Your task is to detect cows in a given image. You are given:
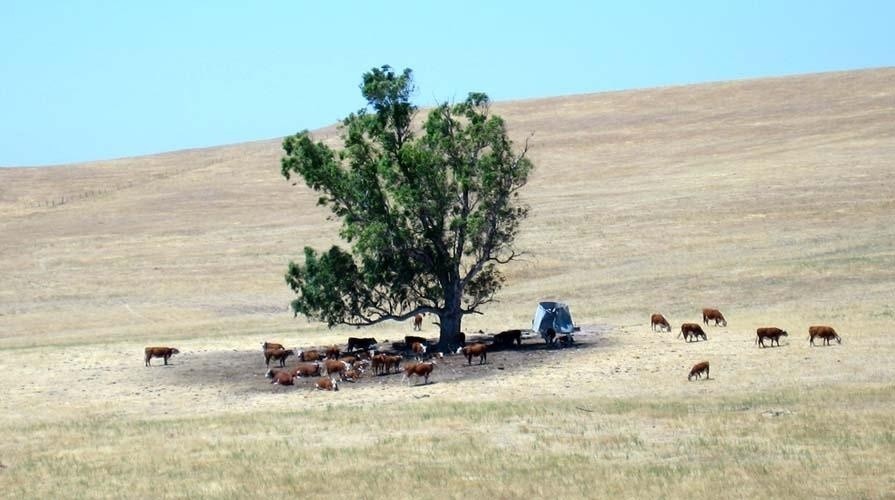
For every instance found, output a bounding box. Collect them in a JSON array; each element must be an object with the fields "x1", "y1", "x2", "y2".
[
  {"x1": 754, "y1": 327, "x2": 788, "y2": 348},
  {"x1": 677, "y1": 323, "x2": 707, "y2": 343},
  {"x1": 687, "y1": 361, "x2": 710, "y2": 382},
  {"x1": 544, "y1": 328, "x2": 555, "y2": 346},
  {"x1": 410, "y1": 341, "x2": 429, "y2": 361},
  {"x1": 401, "y1": 361, "x2": 435, "y2": 386},
  {"x1": 412, "y1": 313, "x2": 423, "y2": 331},
  {"x1": 807, "y1": 326, "x2": 841, "y2": 346},
  {"x1": 259, "y1": 336, "x2": 405, "y2": 390},
  {"x1": 463, "y1": 341, "x2": 488, "y2": 365},
  {"x1": 702, "y1": 308, "x2": 727, "y2": 328},
  {"x1": 144, "y1": 346, "x2": 179, "y2": 368},
  {"x1": 651, "y1": 313, "x2": 672, "y2": 332}
]
